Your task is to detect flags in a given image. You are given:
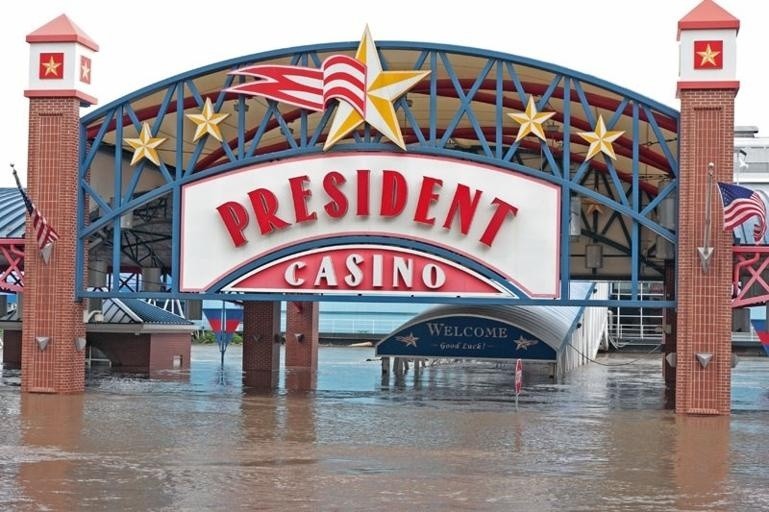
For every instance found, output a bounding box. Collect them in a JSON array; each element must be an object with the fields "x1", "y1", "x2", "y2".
[
  {"x1": 14, "y1": 172, "x2": 59, "y2": 266},
  {"x1": 716, "y1": 182, "x2": 766, "y2": 241}
]
